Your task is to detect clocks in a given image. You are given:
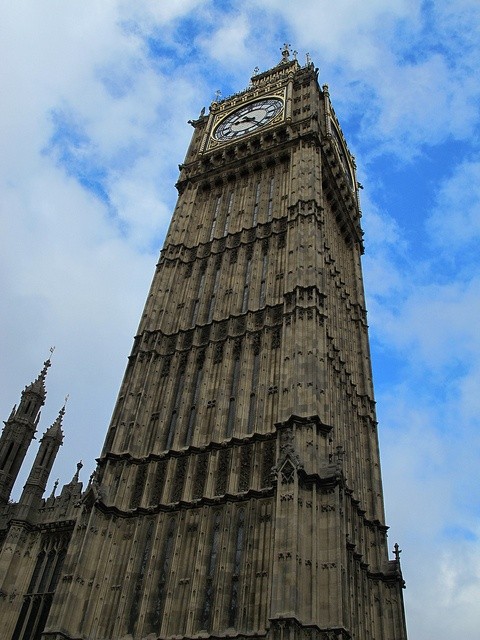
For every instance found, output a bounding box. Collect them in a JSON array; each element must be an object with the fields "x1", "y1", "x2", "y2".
[
  {"x1": 197, "y1": 71, "x2": 294, "y2": 157},
  {"x1": 322, "y1": 83, "x2": 362, "y2": 218}
]
